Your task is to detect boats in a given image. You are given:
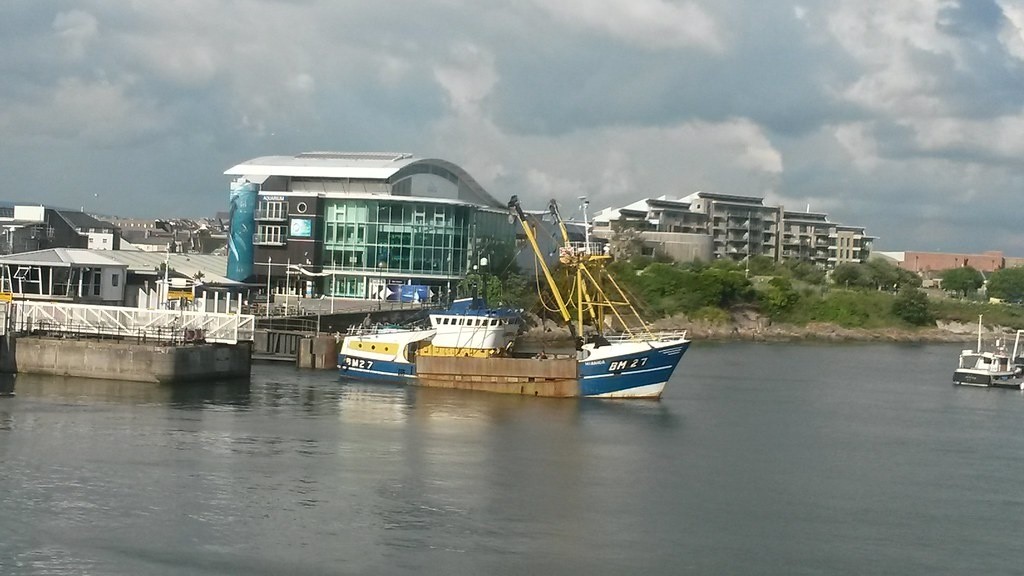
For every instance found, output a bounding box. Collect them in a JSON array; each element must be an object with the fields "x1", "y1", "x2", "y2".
[
  {"x1": 952, "y1": 312, "x2": 1024, "y2": 390},
  {"x1": 337, "y1": 192, "x2": 692, "y2": 399}
]
